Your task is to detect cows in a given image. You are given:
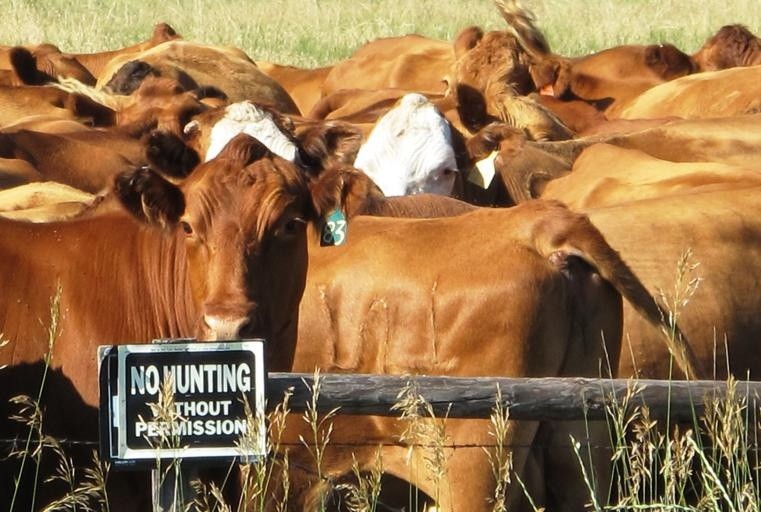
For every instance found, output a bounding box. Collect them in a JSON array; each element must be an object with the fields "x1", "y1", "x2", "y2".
[
  {"x1": 239, "y1": 197, "x2": 705, "y2": 511},
  {"x1": 0, "y1": 2, "x2": 761, "y2": 223},
  {"x1": 526, "y1": 179, "x2": 760, "y2": 512},
  {"x1": 0, "y1": 133, "x2": 334, "y2": 512}
]
